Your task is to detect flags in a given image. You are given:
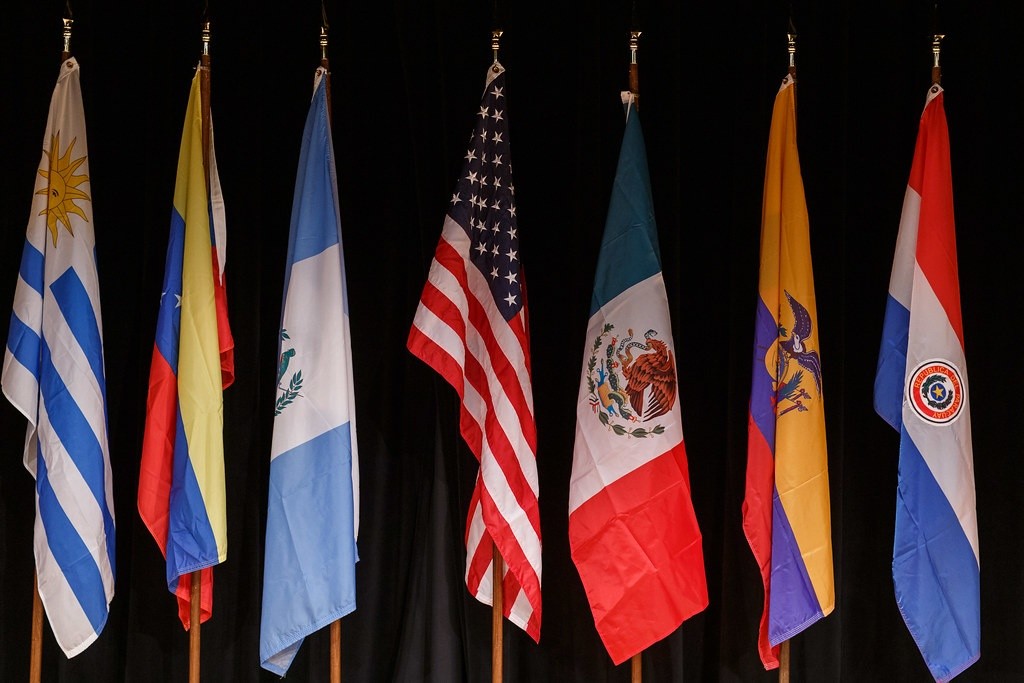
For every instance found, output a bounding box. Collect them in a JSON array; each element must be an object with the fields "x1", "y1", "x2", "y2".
[
  {"x1": 138, "y1": 62, "x2": 235, "y2": 631},
  {"x1": 2, "y1": 58, "x2": 116, "y2": 658},
  {"x1": 873, "y1": 82, "x2": 982, "y2": 683},
  {"x1": 568, "y1": 90, "x2": 708, "y2": 667},
  {"x1": 260, "y1": 67, "x2": 360, "y2": 677},
  {"x1": 741, "y1": 73, "x2": 835, "y2": 671},
  {"x1": 408, "y1": 65, "x2": 542, "y2": 644}
]
